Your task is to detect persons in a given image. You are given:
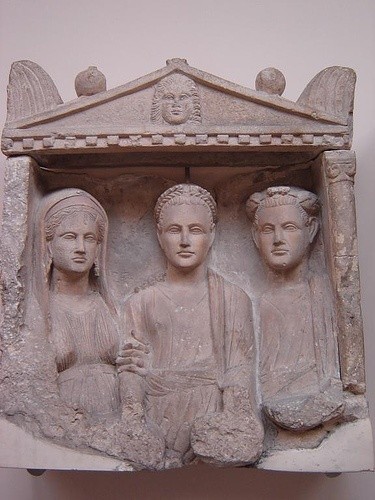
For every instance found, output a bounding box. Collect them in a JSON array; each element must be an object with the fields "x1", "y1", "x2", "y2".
[
  {"x1": 116, "y1": 183, "x2": 257, "y2": 452},
  {"x1": 151, "y1": 73, "x2": 202, "y2": 126},
  {"x1": 30, "y1": 188, "x2": 152, "y2": 419},
  {"x1": 243, "y1": 185, "x2": 340, "y2": 394}
]
